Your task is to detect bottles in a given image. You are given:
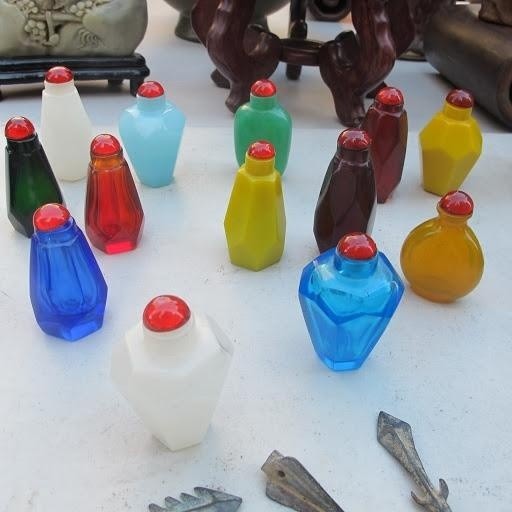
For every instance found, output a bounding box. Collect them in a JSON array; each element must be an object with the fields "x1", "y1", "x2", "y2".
[
  {"x1": 84, "y1": 134, "x2": 145, "y2": 254},
  {"x1": 110, "y1": 294, "x2": 232, "y2": 451},
  {"x1": 5, "y1": 116, "x2": 66, "y2": 240},
  {"x1": 400, "y1": 192, "x2": 486, "y2": 306},
  {"x1": 314, "y1": 127, "x2": 378, "y2": 254},
  {"x1": 417, "y1": 89, "x2": 483, "y2": 196},
  {"x1": 222, "y1": 141, "x2": 286, "y2": 272},
  {"x1": 118, "y1": 80, "x2": 184, "y2": 189},
  {"x1": 362, "y1": 85, "x2": 409, "y2": 205},
  {"x1": 28, "y1": 204, "x2": 108, "y2": 342},
  {"x1": 298, "y1": 232, "x2": 404, "y2": 372},
  {"x1": 234, "y1": 79, "x2": 291, "y2": 178},
  {"x1": 39, "y1": 67, "x2": 98, "y2": 183}
]
